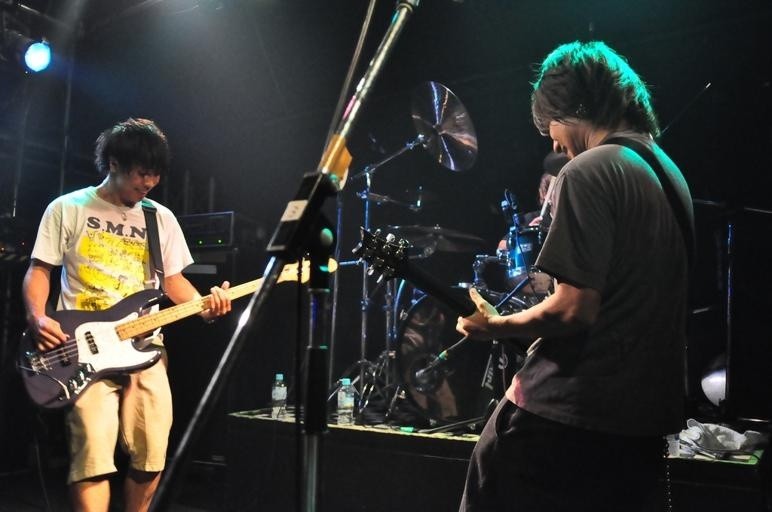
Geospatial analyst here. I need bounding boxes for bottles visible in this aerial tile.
[270,373,288,421]
[337,378,355,427]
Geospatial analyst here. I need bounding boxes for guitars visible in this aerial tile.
[351,226,536,349]
[14,257,337,409]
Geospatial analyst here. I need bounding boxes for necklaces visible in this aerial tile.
[104,181,132,221]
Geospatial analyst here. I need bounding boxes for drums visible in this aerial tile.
[506,225,554,296]
[473,254,506,293]
[397,286,536,429]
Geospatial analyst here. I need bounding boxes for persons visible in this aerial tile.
[455,41,693,512]
[21,118,231,512]
[497,152,570,253]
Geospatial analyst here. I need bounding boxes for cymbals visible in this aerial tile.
[409,79,479,170]
[357,190,410,211]
[396,223,492,242]
